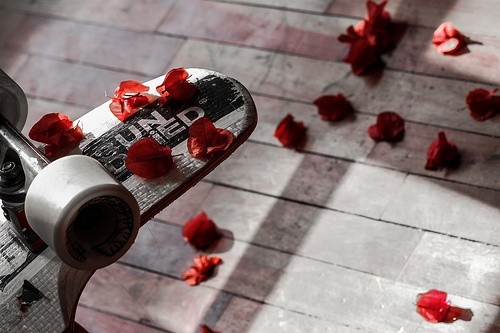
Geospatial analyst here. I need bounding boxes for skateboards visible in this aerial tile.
[0,67,258,333]
